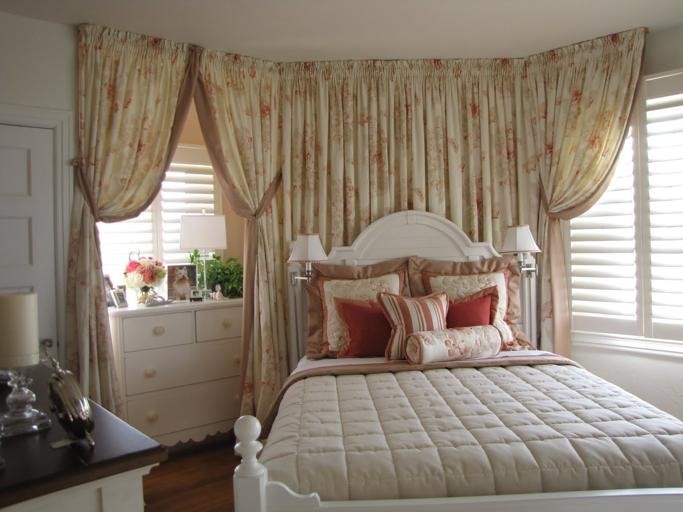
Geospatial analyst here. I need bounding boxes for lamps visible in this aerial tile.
[499,224,542,278]
[180,209,226,300]
[286,234,328,287]
[0,292,52,438]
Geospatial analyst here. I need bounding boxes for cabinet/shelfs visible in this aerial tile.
[107,297,244,460]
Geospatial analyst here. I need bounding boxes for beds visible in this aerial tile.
[233,210,683,512]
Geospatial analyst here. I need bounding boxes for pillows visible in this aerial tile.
[446,285,499,329]
[407,256,535,350]
[307,257,409,360]
[404,324,504,363]
[376,289,449,362]
[334,297,392,360]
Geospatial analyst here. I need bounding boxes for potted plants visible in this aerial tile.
[189,249,243,298]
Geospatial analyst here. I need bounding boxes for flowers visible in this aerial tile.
[124,257,165,293]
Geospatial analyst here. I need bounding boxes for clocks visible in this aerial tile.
[37,345,96,452]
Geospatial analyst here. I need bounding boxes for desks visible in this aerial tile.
[0,362,167,512]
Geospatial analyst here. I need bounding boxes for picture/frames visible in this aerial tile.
[167,264,198,302]
[104,276,128,308]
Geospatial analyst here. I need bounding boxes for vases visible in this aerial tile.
[136,289,152,303]
